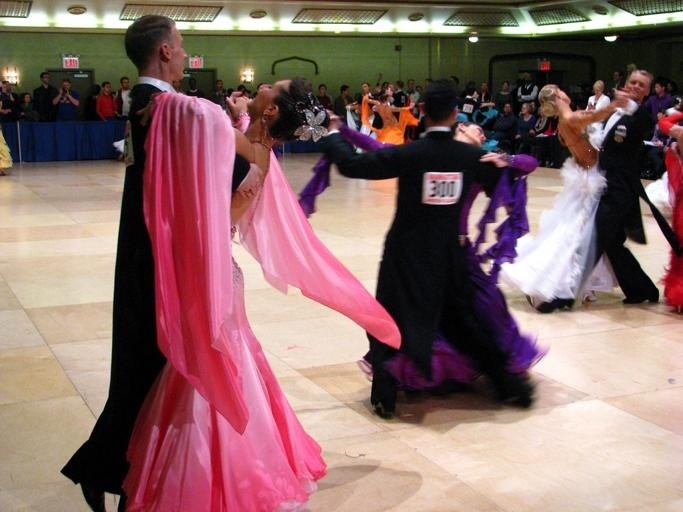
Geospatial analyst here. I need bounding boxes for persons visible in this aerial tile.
[1,65,683,174]
[61,15,250,512]
[123,78,400,512]
[298,81,550,420]
[504,72,683,314]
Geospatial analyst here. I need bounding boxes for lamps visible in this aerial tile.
[604,34,619,42]
[468,32,478,42]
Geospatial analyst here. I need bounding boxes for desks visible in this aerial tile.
[2,120,127,162]
[276,135,339,153]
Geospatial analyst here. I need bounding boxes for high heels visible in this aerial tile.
[582,292,598,304]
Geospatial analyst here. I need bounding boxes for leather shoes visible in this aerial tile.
[61,451,107,512]
[494,389,535,411]
[621,289,659,306]
[373,399,395,421]
[535,298,575,314]
